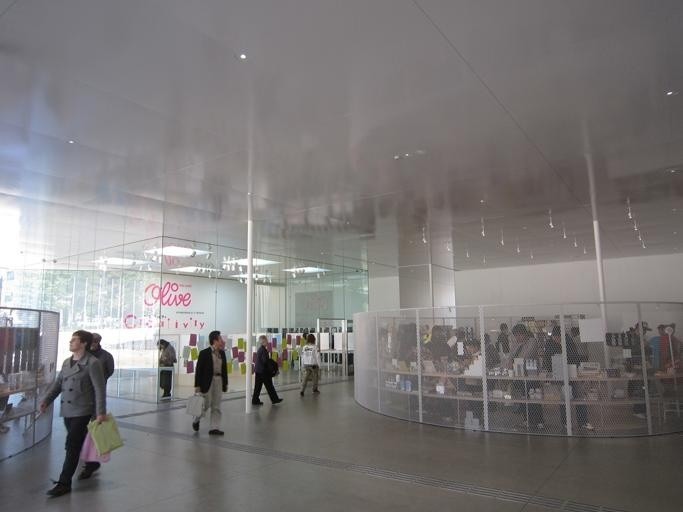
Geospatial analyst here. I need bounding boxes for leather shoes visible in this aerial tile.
[209,430,223,435]
[192,421,198,431]
[272,399,282,404]
[252,401,262,404]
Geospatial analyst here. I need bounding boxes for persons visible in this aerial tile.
[157,339,177,400]
[38,330,108,497]
[88,333,114,422]
[192,331,228,436]
[377,317,593,430]
[252,335,284,406]
[299,334,321,395]
[622,323,683,419]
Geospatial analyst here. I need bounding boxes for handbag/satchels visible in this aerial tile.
[86,413,124,456]
[186,392,205,418]
[80,432,110,463]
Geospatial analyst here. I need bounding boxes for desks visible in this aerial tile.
[114,365,175,404]
[286,349,353,372]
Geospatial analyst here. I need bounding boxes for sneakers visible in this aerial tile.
[632,412,646,419]
[311,390,319,394]
[514,419,593,430]
[160,393,171,400]
[301,391,304,397]
[78,463,99,480]
[45,482,71,495]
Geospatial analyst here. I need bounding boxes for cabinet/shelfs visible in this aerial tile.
[0,376,53,437]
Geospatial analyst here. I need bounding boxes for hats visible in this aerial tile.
[636,322,650,331]
